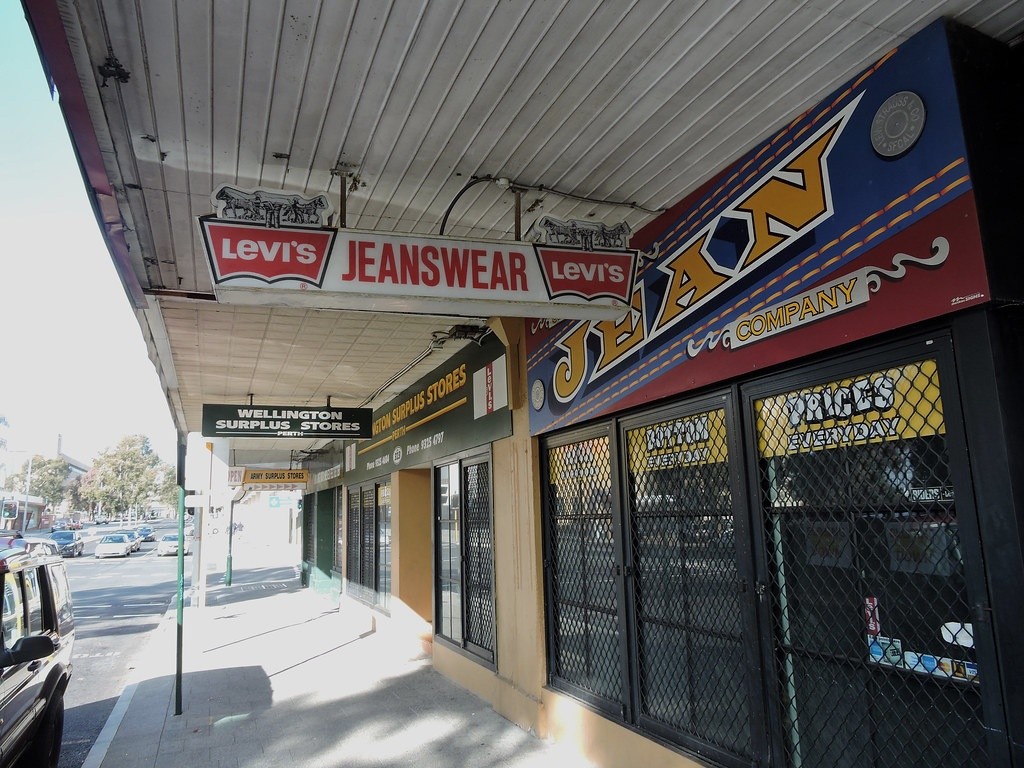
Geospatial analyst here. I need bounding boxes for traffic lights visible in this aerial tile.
[2,501,18,519]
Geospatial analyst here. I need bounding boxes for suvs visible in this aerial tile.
[0,547,76,768]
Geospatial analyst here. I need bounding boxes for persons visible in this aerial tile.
[16,530,22,538]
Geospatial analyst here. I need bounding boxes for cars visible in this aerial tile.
[50,518,84,533]
[148,513,156,519]
[49,531,84,558]
[133,524,156,542]
[664,530,738,589]
[183,526,194,536]
[156,534,191,557]
[124,516,135,521]
[95,534,131,559]
[111,530,142,552]
[113,516,120,522]
[95,516,109,525]
